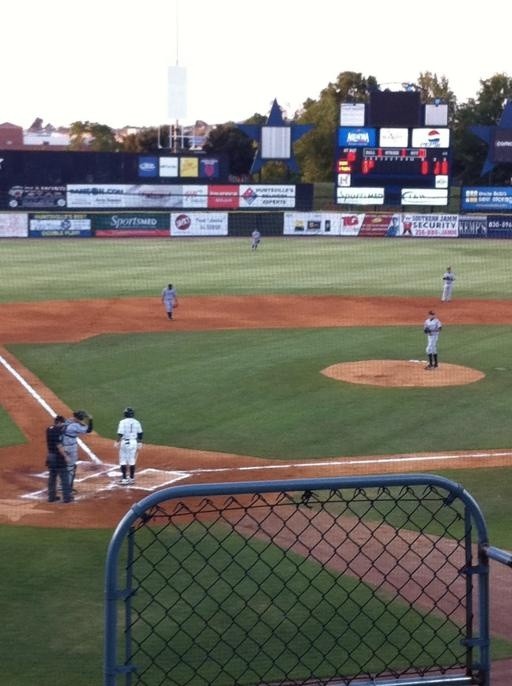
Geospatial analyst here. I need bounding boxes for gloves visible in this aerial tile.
[113,442,119,449]
[137,443,143,450]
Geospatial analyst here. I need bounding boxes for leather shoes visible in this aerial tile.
[48,497,60,502]
[64,496,74,503]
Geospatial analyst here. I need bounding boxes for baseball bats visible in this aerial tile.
[135,449,139,464]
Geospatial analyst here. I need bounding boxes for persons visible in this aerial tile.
[161,284,180,320]
[249,226,261,252]
[401,213,412,236]
[58,410,95,496]
[386,216,400,238]
[45,414,75,504]
[111,406,144,485]
[440,265,456,303]
[423,309,442,370]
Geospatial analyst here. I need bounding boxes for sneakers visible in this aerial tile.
[434,364,439,369]
[128,479,135,484]
[424,364,433,370]
[117,479,128,486]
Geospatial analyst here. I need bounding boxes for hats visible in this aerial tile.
[55,415,69,426]
[429,310,435,315]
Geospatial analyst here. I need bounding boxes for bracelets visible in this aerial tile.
[62,454,68,459]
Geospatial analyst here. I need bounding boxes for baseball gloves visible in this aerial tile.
[172,302,179,309]
[425,327,430,331]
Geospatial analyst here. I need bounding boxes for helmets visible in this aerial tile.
[123,407,135,418]
[73,409,89,420]
